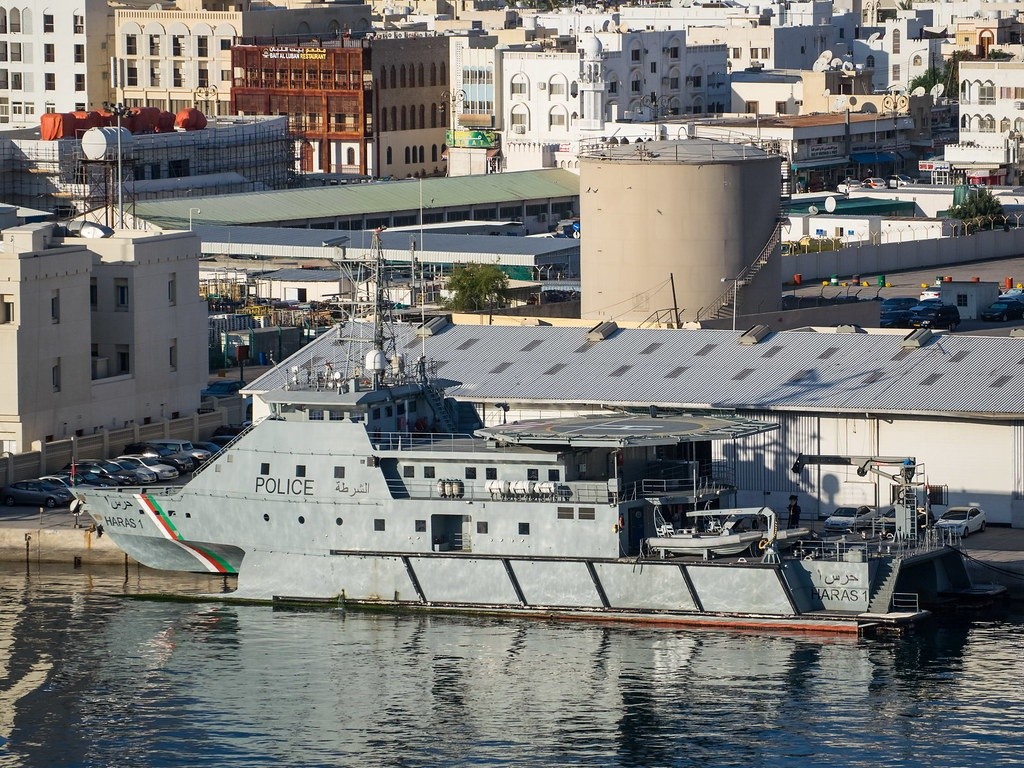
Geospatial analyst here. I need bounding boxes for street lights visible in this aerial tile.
[437,88,468,146]
[639,94,673,141]
[194,85,221,117]
[879,90,909,189]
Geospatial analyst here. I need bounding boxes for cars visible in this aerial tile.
[934,507,986,539]
[824,505,877,533]
[836,174,917,196]
[981,288,1024,322]
[0,421,252,509]
[874,507,935,539]
[200,380,247,403]
[880,286,962,329]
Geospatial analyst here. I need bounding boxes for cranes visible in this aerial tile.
[790,452,925,541]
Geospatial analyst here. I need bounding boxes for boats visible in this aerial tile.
[67,224,976,643]
[645,531,763,555]
